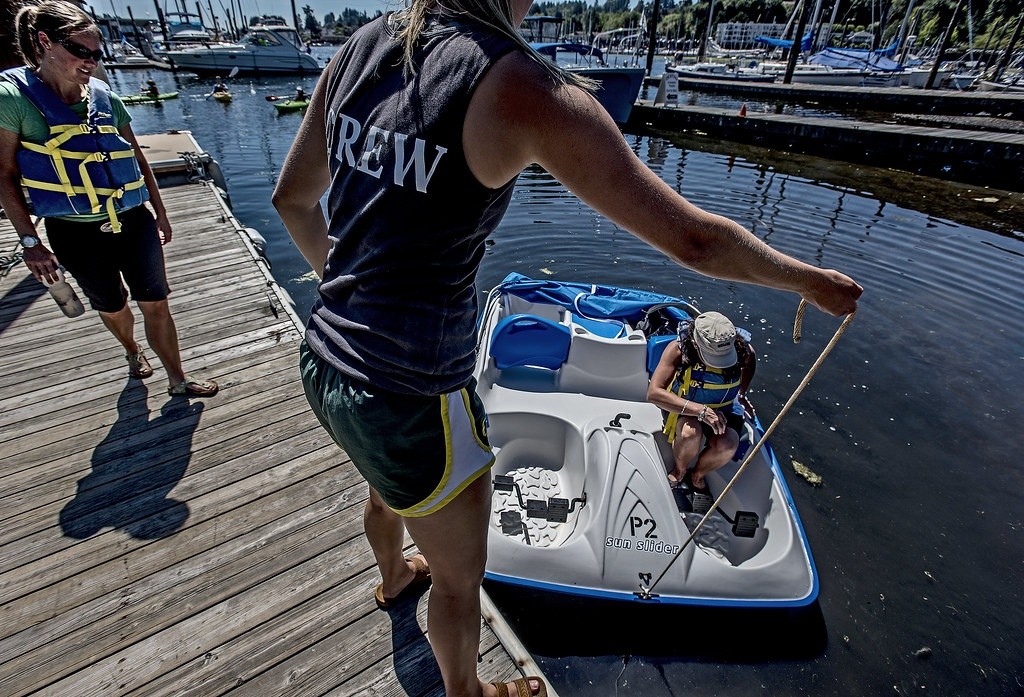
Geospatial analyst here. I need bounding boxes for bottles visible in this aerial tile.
[38,261,87,320]
[740,104,746,116]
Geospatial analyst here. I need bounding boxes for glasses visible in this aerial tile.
[61,40,104,60]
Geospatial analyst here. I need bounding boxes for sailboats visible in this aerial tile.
[664,0,1024,91]
[118,0,312,62]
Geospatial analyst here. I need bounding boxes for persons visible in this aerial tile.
[0,0,221,398]
[212,76,229,93]
[253,34,269,46]
[293,86,309,102]
[270,0,864,697]
[646,312,756,490]
[141,79,159,97]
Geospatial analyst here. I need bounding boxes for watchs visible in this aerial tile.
[20,234,40,247]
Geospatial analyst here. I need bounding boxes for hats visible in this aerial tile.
[693,311,737,368]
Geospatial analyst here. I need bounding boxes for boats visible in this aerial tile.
[211,91,234,103]
[163,27,327,76]
[273,97,310,112]
[120,91,179,103]
[516,16,646,125]
[472,271,821,609]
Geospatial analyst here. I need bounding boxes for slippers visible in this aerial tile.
[689,471,710,500]
[491,676,547,697]
[375,550,431,606]
[125,343,152,379]
[167,375,219,396]
[667,476,685,488]
